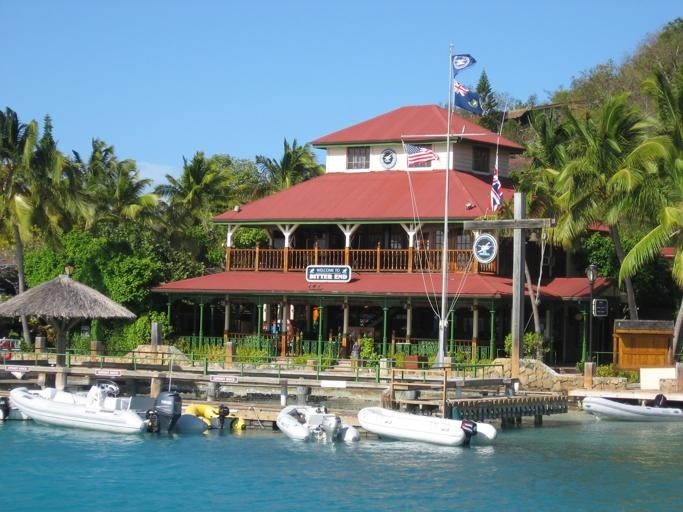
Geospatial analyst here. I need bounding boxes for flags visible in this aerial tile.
[490,135,505,213]
[453,79,485,114]
[405,143,440,167]
[452,54,477,79]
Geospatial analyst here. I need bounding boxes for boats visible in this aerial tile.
[275,405,361,447]
[358,407,498,445]
[358,436,496,460]
[0,397,29,422]
[8,381,208,436]
[186,403,245,434]
[582,393,682,425]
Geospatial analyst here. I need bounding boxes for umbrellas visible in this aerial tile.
[0,268,137,390]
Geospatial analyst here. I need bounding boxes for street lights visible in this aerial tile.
[584,263,597,379]
[64,263,74,352]
